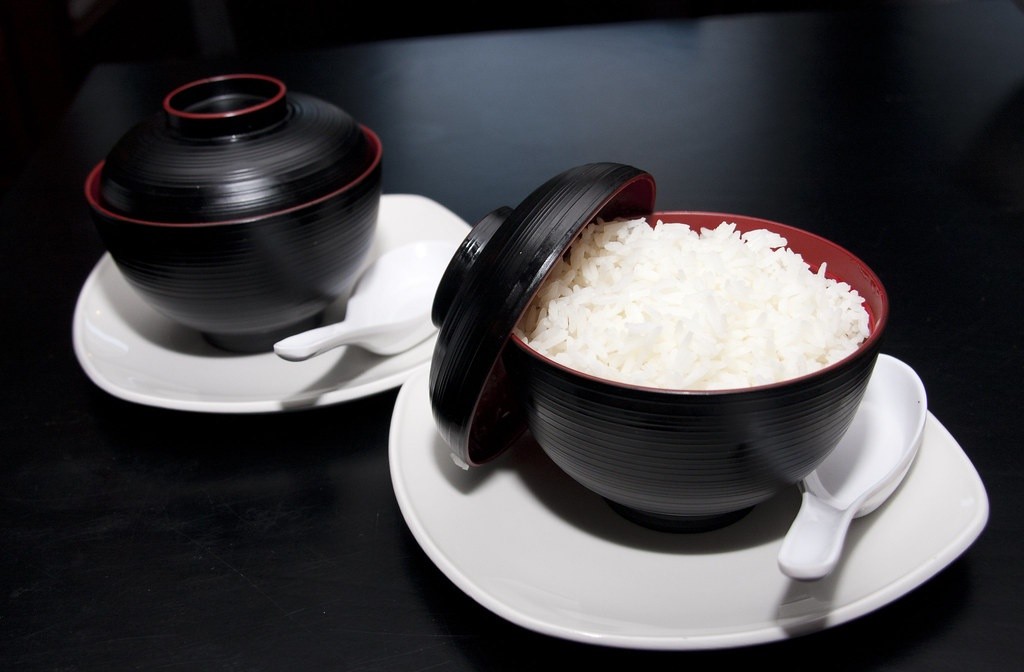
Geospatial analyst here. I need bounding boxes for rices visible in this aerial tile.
[511,211,871,392]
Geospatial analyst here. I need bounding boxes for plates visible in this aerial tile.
[75,194,478,410]
[388,359,992,650]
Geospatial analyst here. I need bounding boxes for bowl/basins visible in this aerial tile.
[85,71,381,343]
[507,210,887,518]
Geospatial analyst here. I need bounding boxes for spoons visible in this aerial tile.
[272,242,454,360]
[777,352,929,579]
